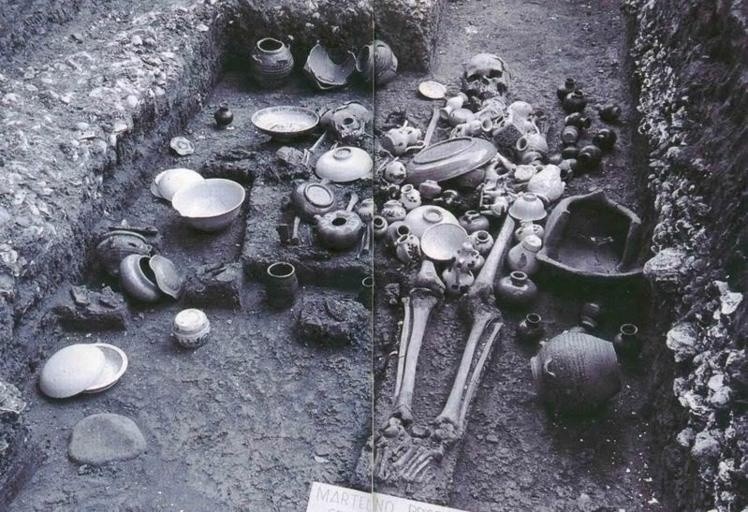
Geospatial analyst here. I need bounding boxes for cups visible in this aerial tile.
[267,262,299,308]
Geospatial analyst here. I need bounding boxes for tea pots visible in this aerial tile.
[382,120,427,155]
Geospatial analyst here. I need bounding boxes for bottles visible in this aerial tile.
[247,37,296,91]
[360,162,443,310]
[354,38,400,86]
[213,101,233,131]
[443,77,641,409]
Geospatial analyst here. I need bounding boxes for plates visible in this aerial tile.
[407,137,501,185]
[418,80,445,99]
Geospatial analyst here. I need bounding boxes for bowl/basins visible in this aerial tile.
[151,167,203,201]
[82,342,129,393]
[94,230,150,277]
[170,179,246,234]
[41,343,104,398]
[314,146,372,183]
[120,254,162,303]
[295,182,338,222]
[423,220,471,262]
[403,203,458,241]
[251,105,320,141]
